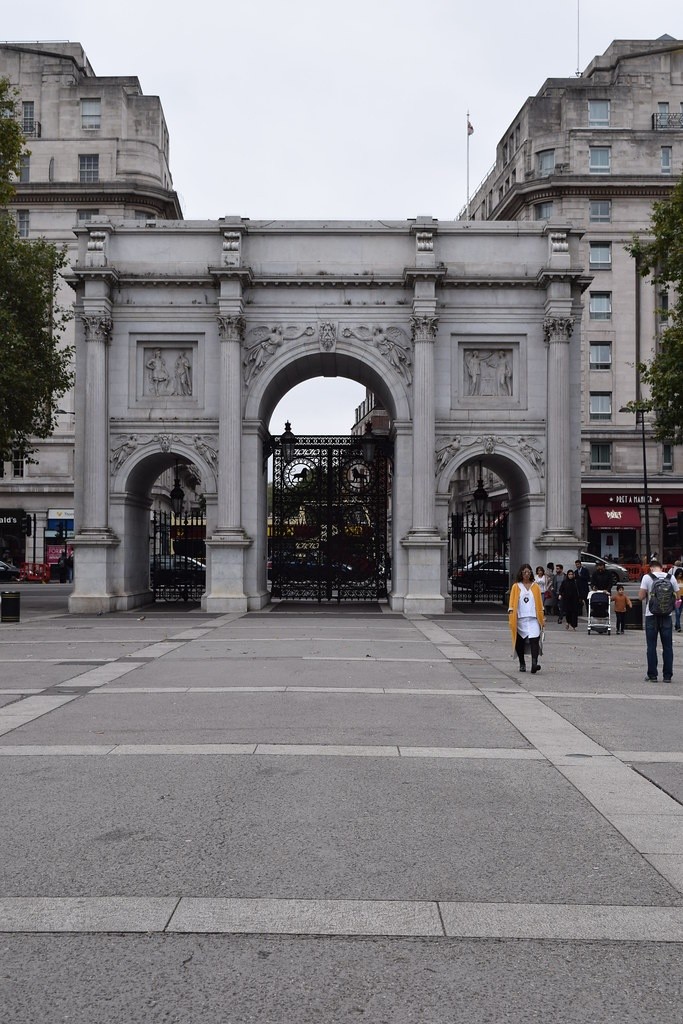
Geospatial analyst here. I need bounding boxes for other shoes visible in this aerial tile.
[663,677,671,683]
[531,665,540,673]
[676,628,681,632]
[566,622,568,629]
[616,628,621,635]
[557,618,562,624]
[572,627,575,632]
[621,627,624,634]
[644,674,657,682]
[519,664,526,671]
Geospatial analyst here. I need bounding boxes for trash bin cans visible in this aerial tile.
[624,599,644,628]
[1,591,21,622]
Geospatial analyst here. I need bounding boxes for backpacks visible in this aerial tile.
[648,572,675,616]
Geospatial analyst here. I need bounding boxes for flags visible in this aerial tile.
[468,121,475,137]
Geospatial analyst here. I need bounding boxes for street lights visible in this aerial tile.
[618,406,654,565]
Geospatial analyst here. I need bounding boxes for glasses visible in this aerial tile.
[521,570,531,574]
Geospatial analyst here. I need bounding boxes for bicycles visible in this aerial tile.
[159,563,205,605]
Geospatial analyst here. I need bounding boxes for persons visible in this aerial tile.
[590,562,613,635]
[482,435,497,454]
[502,436,543,475]
[349,325,411,386]
[469,350,483,395]
[535,560,591,632]
[435,434,461,476]
[508,563,547,673]
[489,349,511,396]
[319,321,336,351]
[159,434,170,451]
[604,552,683,683]
[611,586,632,634]
[110,434,152,475]
[174,351,191,396]
[244,324,308,386]
[477,551,501,560]
[193,434,219,477]
[146,349,167,396]
[58,549,74,583]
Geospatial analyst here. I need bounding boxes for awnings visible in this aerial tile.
[588,507,683,530]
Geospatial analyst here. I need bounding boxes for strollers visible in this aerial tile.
[586,589,612,636]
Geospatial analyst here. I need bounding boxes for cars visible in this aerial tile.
[0,559,20,582]
[449,558,509,593]
[581,551,630,587]
[150,554,207,587]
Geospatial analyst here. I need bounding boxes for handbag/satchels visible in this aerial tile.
[675,599,682,608]
[545,591,551,599]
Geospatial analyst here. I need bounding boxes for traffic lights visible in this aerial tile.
[22,514,32,537]
[54,521,64,541]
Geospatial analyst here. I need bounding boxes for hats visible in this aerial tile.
[595,560,607,565]
